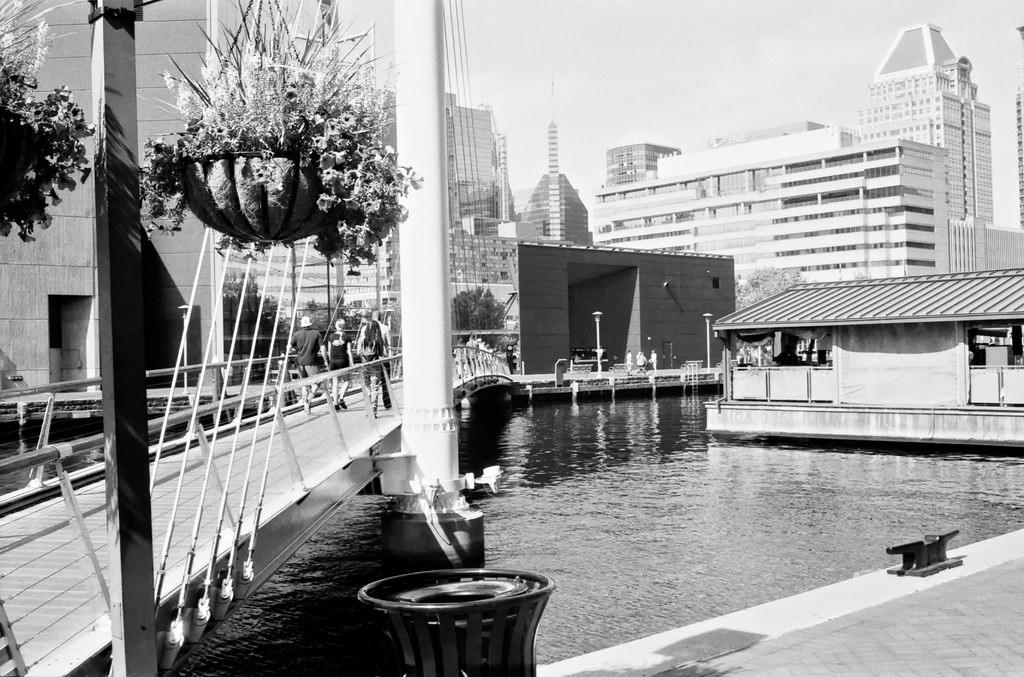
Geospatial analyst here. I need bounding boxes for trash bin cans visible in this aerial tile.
[357,568,557,677]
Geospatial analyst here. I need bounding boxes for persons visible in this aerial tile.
[465,336,479,376]
[626,351,633,376]
[290,316,328,415]
[327,318,353,410]
[772,345,798,367]
[358,320,383,418]
[454,338,465,377]
[636,352,647,372]
[356,311,392,410]
[476,338,493,373]
[651,350,657,373]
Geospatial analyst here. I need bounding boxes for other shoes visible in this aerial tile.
[365,414,378,419]
[304,399,312,416]
[338,402,348,409]
[335,404,341,411]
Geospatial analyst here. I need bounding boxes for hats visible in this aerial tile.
[300,316,314,329]
